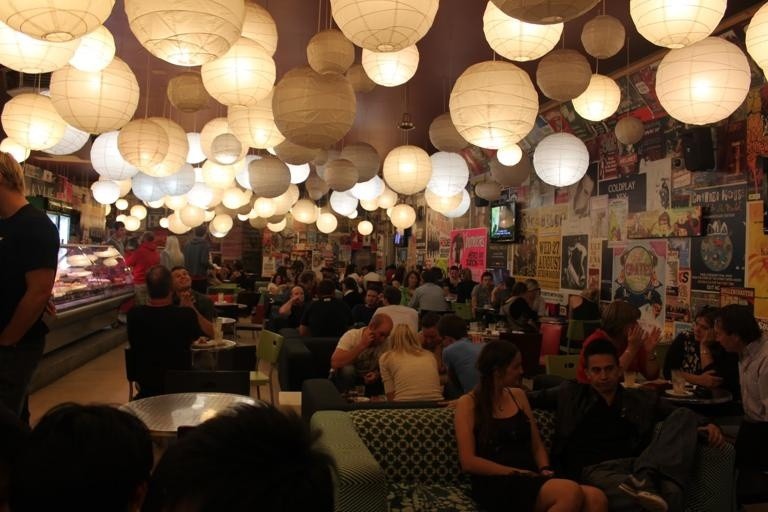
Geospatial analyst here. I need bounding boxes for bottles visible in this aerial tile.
[488,308,495,331]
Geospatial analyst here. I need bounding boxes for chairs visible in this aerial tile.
[124,345,136,402]
[250,330,284,407]
[452,302,473,321]
[560,319,603,355]
[544,354,580,383]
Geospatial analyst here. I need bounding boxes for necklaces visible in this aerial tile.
[492,394,508,416]
[699,348,711,355]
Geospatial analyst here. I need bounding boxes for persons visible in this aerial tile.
[384,264,395,287]
[29,404,151,512]
[578,300,663,391]
[342,278,364,305]
[443,264,460,290]
[525,343,725,512]
[140,401,341,512]
[521,277,548,315]
[351,287,384,324]
[329,311,393,397]
[379,323,446,400]
[109,221,128,248]
[668,304,728,393]
[276,256,303,278]
[321,255,336,282]
[421,319,451,371]
[493,276,515,305]
[413,264,424,285]
[117,229,162,303]
[186,225,211,281]
[449,342,607,512]
[162,237,187,276]
[431,266,448,288]
[438,314,492,399]
[363,264,383,290]
[568,285,604,346]
[264,270,290,296]
[471,270,496,317]
[0,147,61,512]
[167,266,218,343]
[502,285,527,326]
[457,267,480,303]
[715,301,768,510]
[371,285,418,338]
[399,271,418,306]
[271,286,306,327]
[391,265,405,285]
[307,280,351,337]
[232,263,246,286]
[409,270,446,313]
[128,265,206,396]
[298,267,318,296]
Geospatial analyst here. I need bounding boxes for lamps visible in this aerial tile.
[475,181,501,201]
[286,162,310,184]
[324,159,359,192]
[202,159,236,189]
[254,197,277,218]
[235,155,262,190]
[745,2,768,83]
[39,91,90,156]
[205,192,222,209]
[497,144,523,166]
[358,221,373,236]
[630,0,728,49]
[339,142,381,184]
[360,199,378,211]
[449,60,539,150]
[249,157,291,198]
[330,190,359,216]
[425,187,464,211]
[244,190,253,205]
[615,117,644,145]
[345,62,376,94]
[383,145,432,196]
[227,85,276,148]
[386,207,394,217]
[213,214,234,232]
[348,209,358,219]
[168,214,188,235]
[581,14,625,59]
[655,36,752,126]
[305,173,330,201]
[185,132,206,164]
[267,214,285,224]
[429,112,470,153]
[249,216,267,229]
[125,216,141,232]
[306,28,355,74]
[155,164,196,197]
[316,213,338,234]
[164,195,187,211]
[193,168,203,183]
[536,48,593,101]
[187,183,215,208]
[265,126,286,156]
[0,0,116,43]
[268,217,287,233]
[90,130,139,181]
[390,204,417,230]
[49,56,140,135]
[116,214,127,225]
[1,92,66,151]
[271,65,357,149]
[200,117,249,165]
[139,117,190,178]
[220,203,236,219]
[287,184,300,206]
[130,204,147,220]
[273,139,322,165]
[490,151,530,187]
[249,209,257,219]
[362,43,420,87]
[237,213,249,221]
[209,220,229,238]
[483,1,564,62]
[305,205,319,224]
[572,74,621,122]
[115,199,128,210]
[292,199,314,222]
[238,203,251,215]
[311,149,328,165]
[93,180,120,204]
[330,0,440,53]
[0,137,31,163]
[148,200,164,209]
[201,36,277,110]
[198,208,216,222]
[221,187,244,209]
[490,0,601,25]
[124,0,246,67]
[316,150,342,182]
[159,217,168,228]
[132,172,164,202]
[533,132,590,187]
[350,176,385,200]
[101,204,111,216]
[242,2,279,57]
[378,184,397,209]
[0,20,81,74]
[439,188,471,218]
[69,25,116,72]
[428,150,470,198]
[117,118,169,169]
[167,72,209,114]
[179,204,205,228]
[211,133,242,165]
[99,175,132,197]
[274,190,292,215]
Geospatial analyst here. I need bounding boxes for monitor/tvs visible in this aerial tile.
[490,202,517,243]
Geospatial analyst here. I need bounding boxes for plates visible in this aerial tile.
[665,389,693,396]
[620,383,641,388]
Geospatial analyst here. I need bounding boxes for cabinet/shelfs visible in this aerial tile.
[29,245,134,393]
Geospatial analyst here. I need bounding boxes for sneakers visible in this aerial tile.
[619,473,668,511]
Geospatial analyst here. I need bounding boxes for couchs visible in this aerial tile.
[301,378,448,426]
[311,407,556,512]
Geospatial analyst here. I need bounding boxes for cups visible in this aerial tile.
[212,317,223,334]
[214,332,224,348]
[624,371,636,387]
[470,322,477,332]
[489,324,495,334]
[672,371,686,394]
[218,293,224,304]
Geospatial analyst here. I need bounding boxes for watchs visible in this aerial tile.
[539,465,552,471]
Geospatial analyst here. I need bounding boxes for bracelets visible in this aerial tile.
[625,349,634,360]
